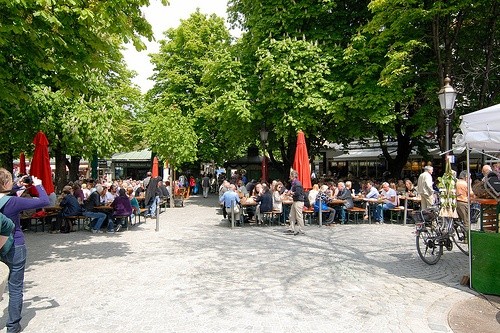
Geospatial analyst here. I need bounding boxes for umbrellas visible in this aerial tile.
[293,130,313,191]
[19,152,27,175]
[29,129,55,195]
[151,157,158,178]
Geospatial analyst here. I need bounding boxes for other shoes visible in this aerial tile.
[294,231,304,236]
[116,225,122,232]
[48,229,61,234]
[105,229,115,233]
[84,225,92,231]
[91,229,104,234]
[283,229,294,233]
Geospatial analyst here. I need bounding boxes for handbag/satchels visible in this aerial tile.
[410,207,436,222]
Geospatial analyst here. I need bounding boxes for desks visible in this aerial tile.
[397,195,421,201]
[357,197,380,222]
[44,205,64,232]
[240,197,259,225]
[94,204,114,227]
[332,198,347,222]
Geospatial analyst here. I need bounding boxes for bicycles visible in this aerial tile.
[414,207,469,265]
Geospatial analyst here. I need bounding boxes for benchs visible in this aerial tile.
[20,209,149,232]
[263,206,416,226]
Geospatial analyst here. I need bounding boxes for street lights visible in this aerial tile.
[258,120,269,183]
[436,73,459,174]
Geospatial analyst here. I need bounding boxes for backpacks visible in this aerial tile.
[0,195,16,258]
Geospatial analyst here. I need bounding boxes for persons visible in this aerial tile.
[440,163,500,244]
[418,166,435,211]
[218,168,418,225]
[286,169,306,235]
[10,168,188,234]
[0,167,51,333]
[190,171,227,198]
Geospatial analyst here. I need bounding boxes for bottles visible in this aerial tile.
[49,192,56,206]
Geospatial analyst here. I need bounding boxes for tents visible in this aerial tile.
[453,104,500,289]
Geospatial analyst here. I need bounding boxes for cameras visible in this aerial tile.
[23,176,33,183]
